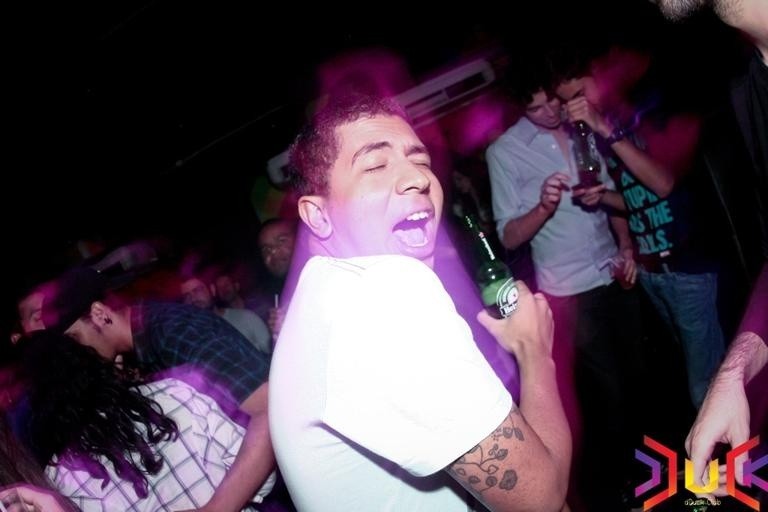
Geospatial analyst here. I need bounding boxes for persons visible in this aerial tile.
[485,77,638,452]
[545,42,725,411]
[269,90,575,512]
[655,0,768,507]
[275,70,486,328]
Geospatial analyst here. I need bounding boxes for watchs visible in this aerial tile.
[604,128,623,149]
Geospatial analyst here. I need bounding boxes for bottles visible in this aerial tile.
[569,121,600,192]
[462,215,519,319]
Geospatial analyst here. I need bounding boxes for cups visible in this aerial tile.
[0,487,27,511]
